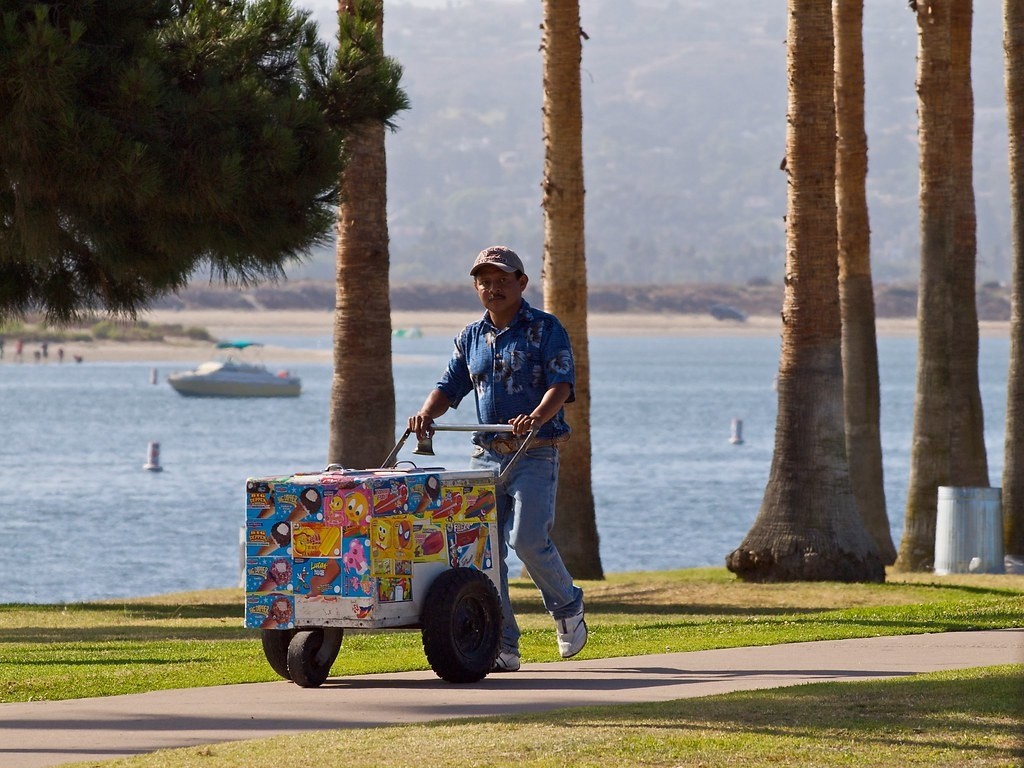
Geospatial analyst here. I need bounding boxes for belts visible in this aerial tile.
[492,434,570,454]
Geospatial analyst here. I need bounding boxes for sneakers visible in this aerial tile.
[555,599,588,657]
[490,652,520,672]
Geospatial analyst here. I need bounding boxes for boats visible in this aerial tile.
[165,341,305,400]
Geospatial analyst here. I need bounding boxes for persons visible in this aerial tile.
[0,338,83,363]
[408,247,588,671]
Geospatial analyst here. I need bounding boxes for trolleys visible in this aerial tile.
[241,421,539,689]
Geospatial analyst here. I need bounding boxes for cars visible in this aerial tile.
[709,304,745,324]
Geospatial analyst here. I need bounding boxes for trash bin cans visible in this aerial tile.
[933,487,1006,574]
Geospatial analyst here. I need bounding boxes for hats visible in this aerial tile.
[469,246,524,275]
